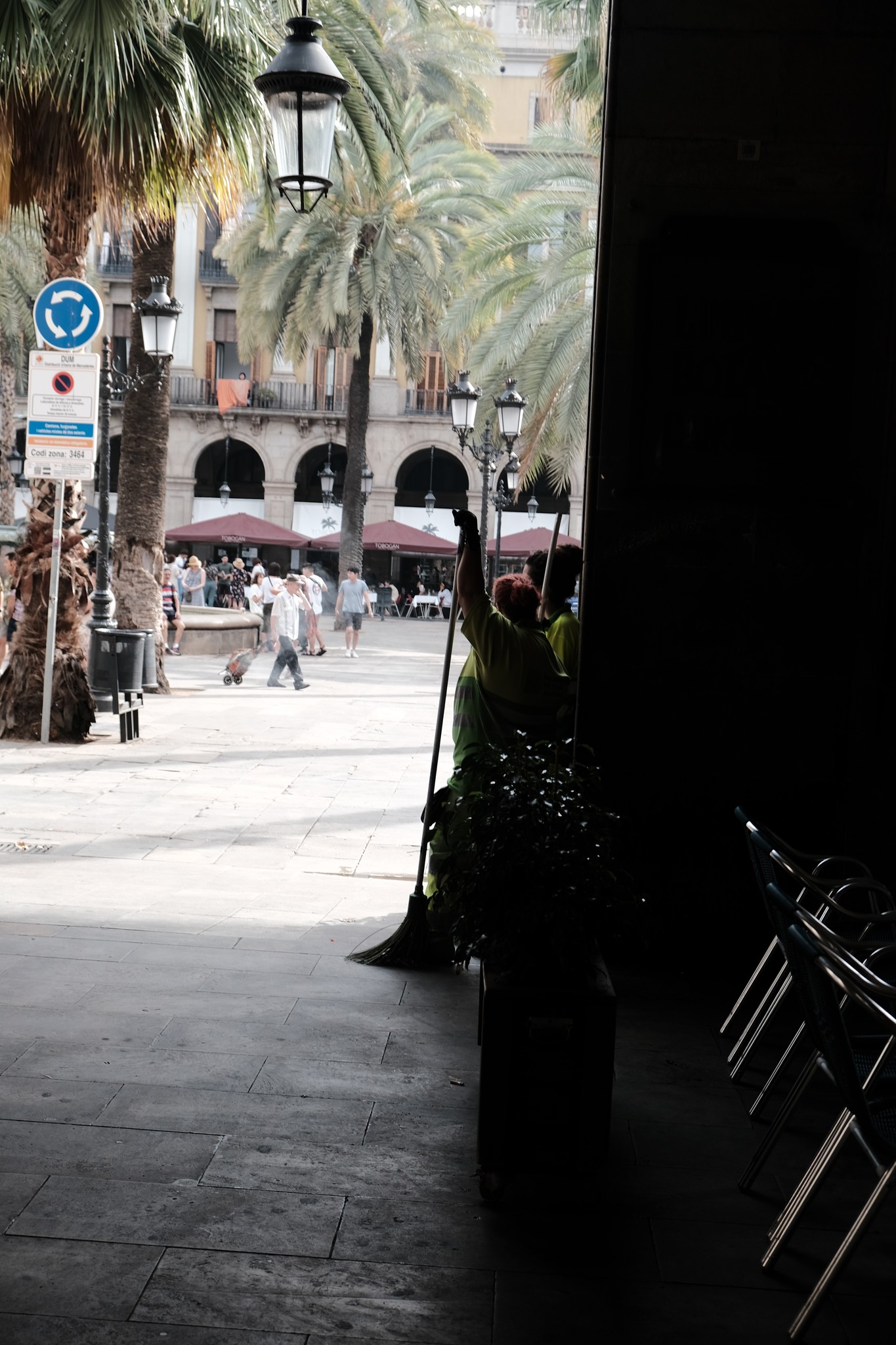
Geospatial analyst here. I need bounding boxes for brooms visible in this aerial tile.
[344,504,472,976]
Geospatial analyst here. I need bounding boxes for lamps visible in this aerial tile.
[527,483,539,523]
[253,0,352,214]
[219,434,231,507]
[317,432,337,512]
[424,444,436,517]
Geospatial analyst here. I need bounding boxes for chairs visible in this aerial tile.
[400,594,417,617]
[391,594,401,617]
[720,805,896,1338]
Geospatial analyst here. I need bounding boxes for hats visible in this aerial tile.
[286,573,305,585]
[187,555,199,566]
[233,558,245,570]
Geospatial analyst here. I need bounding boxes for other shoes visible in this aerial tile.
[170,646,181,655]
[293,680,310,690]
[267,679,286,687]
[345,647,350,657]
[351,650,358,658]
[165,647,172,655]
[298,648,308,653]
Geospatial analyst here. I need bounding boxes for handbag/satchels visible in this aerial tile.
[184,592,192,603]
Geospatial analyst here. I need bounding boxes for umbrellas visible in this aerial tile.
[161,511,309,562]
[309,520,457,598]
[479,525,584,558]
[10,498,117,535]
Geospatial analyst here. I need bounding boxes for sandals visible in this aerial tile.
[316,645,327,656]
[302,649,315,656]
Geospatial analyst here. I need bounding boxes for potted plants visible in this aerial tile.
[256,387,274,408]
[421,728,627,1202]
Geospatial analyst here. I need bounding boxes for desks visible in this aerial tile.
[363,593,380,616]
[405,594,445,619]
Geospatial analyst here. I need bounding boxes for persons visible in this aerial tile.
[0,550,21,674]
[406,578,425,618]
[160,548,399,658]
[266,573,312,691]
[334,566,374,658]
[433,581,452,618]
[237,372,256,407]
[181,555,206,607]
[423,506,585,920]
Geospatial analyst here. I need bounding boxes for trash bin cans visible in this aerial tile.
[90,627,146,694]
[136,628,159,688]
